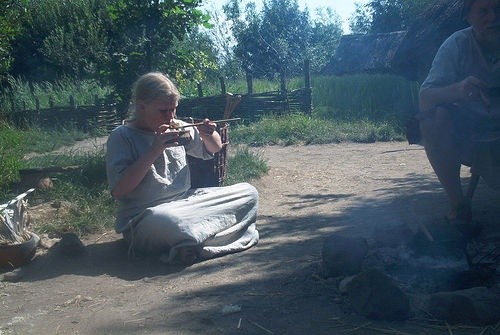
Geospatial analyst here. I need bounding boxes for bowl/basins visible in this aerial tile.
[480,87,500,104]
[162,130,192,145]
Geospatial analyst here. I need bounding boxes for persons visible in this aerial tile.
[105,72,259,272]
[416,1,500,230]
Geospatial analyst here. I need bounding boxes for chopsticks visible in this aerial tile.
[171,118,240,129]
